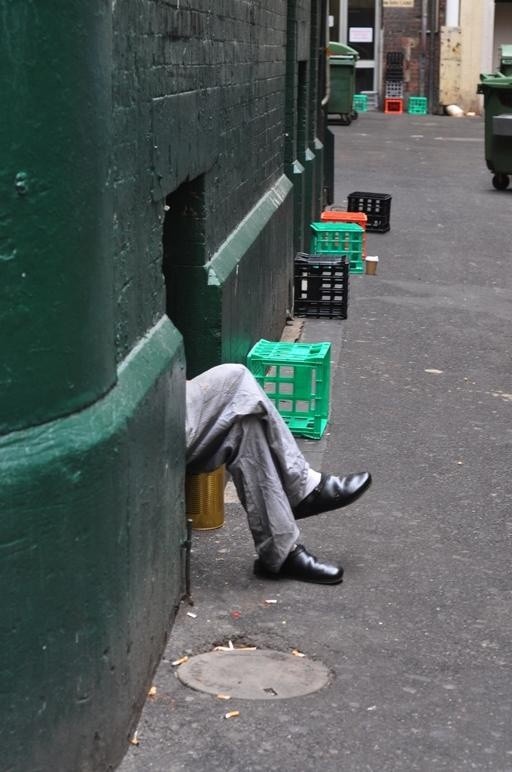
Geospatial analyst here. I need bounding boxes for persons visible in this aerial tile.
[185,362,371,584]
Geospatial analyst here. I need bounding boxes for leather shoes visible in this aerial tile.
[294,473,368,518]
[286,545,344,585]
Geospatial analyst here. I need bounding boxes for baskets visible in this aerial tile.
[354,94,368,112]
[293,250,348,321]
[348,192,392,233]
[383,53,404,114]
[312,210,368,275]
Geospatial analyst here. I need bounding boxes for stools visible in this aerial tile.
[384,51,404,115]
[292,191,392,319]
[353,91,379,112]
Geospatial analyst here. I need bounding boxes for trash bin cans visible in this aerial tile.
[497,43,512,76]
[477,72,512,189]
[327,41,360,125]
